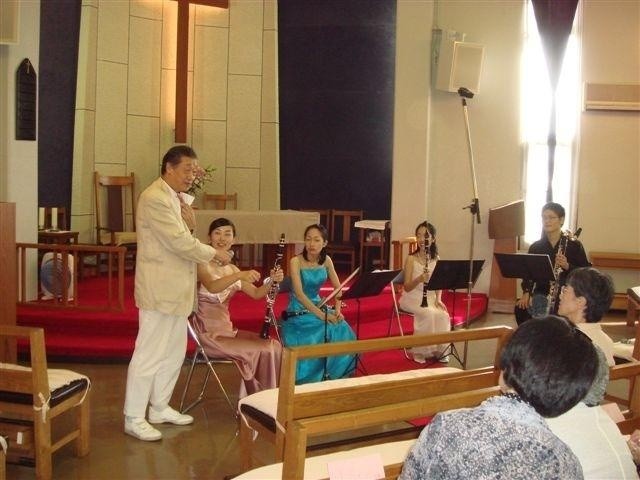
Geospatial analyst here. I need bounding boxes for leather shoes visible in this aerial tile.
[414,354,426,364]
[437,354,449,363]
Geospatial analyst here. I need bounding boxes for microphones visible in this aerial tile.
[458,87,474,99]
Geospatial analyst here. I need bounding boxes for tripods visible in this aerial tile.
[336,300,368,378]
[433,289,464,367]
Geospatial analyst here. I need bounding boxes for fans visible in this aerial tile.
[40,250,76,303]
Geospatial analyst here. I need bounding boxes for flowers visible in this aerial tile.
[186,164,217,196]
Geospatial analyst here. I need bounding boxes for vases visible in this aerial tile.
[180,192,195,205]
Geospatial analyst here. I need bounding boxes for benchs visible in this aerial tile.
[625,285,640,326]
[233,325,639,480]
[0,437,9,480]
[1,323,92,478]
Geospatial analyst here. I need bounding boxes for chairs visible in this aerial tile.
[320,209,364,275]
[177,309,241,419]
[92,170,137,279]
[385,270,437,362]
[202,192,237,211]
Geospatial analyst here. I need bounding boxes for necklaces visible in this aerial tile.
[417,251,432,265]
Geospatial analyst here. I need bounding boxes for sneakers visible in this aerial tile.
[148,406,194,426]
[124,416,163,442]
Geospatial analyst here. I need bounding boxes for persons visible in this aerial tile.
[398,223,451,364]
[192,218,284,399]
[397,315,599,480]
[515,203,589,326]
[558,267,616,367]
[279,224,358,384]
[123,146,234,441]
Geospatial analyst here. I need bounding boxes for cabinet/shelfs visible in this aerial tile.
[38,206,79,244]
[354,219,390,274]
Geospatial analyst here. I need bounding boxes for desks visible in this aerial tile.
[194,210,320,279]
[17,243,126,311]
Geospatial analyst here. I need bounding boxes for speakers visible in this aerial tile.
[436,40,486,95]
